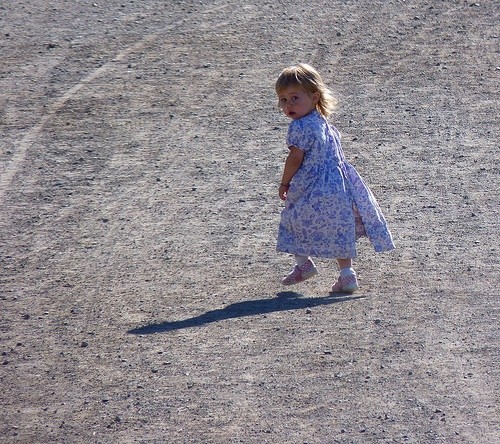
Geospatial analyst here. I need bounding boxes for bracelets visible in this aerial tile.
[281,183,289,187]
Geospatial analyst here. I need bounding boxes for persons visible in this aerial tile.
[275,63,396,293]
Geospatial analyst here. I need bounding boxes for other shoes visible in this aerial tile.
[332,274,359,293]
[281,259,319,285]
